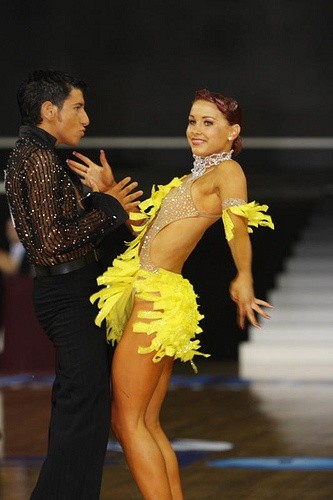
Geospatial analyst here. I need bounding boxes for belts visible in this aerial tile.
[35,249,99,278]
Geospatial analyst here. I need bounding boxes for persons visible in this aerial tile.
[67,87,274,500]
[5,79,143,500]
